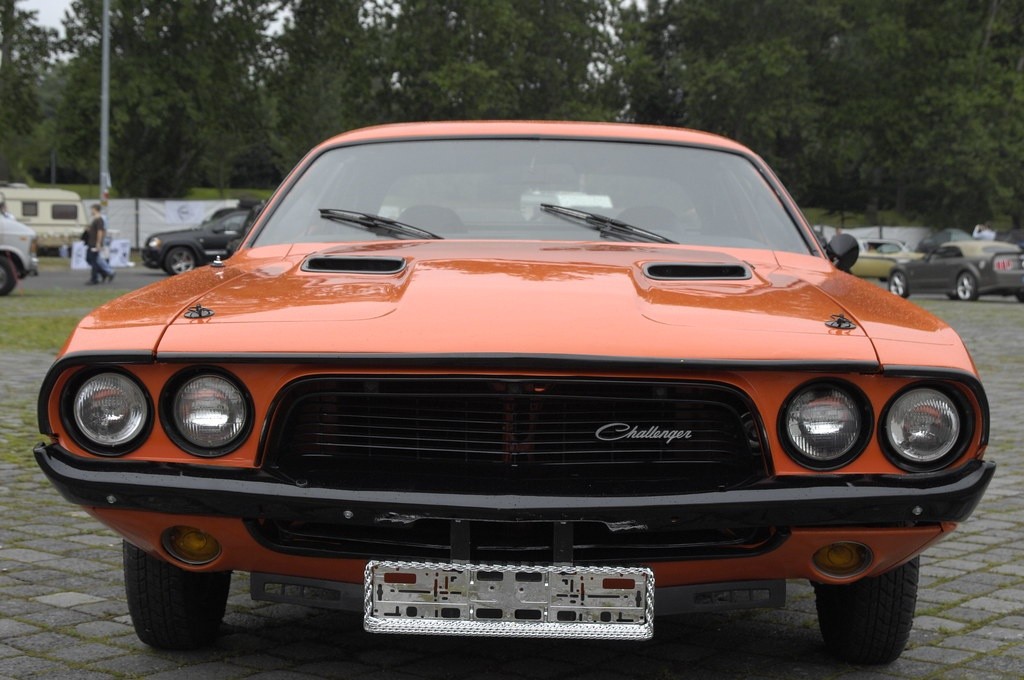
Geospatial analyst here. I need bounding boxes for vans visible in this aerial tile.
[0,181,90,258]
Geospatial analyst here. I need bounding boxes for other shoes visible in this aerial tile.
[85,281,99,285]
[110,272,116,282]
[102,272,108,283]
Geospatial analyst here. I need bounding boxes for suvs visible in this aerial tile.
[141,201,264,277]
[1,205,39,299]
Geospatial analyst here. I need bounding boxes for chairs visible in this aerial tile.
[614,204,686,242]
[393,204,468,236]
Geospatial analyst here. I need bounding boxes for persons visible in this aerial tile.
[972,222,996,241]
[85,204,115,285]
[831,227,842,238]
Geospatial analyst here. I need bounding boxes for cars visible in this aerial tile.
[849,236,936,282]
[887,237,1024,303]
[914,225,1024,257]
[31,118,999,666]
